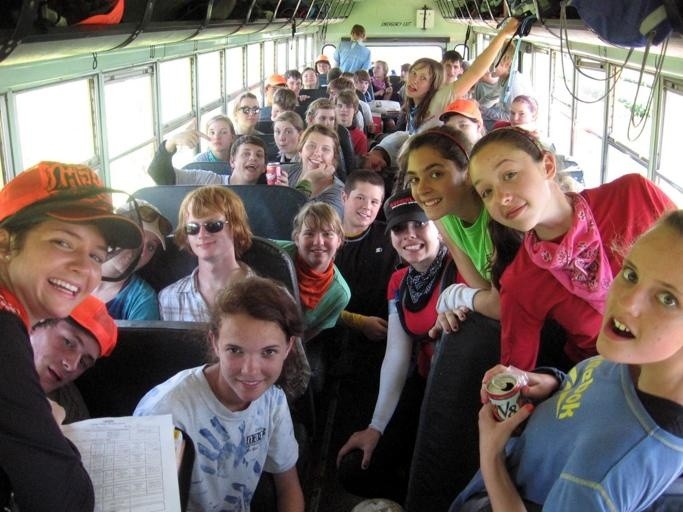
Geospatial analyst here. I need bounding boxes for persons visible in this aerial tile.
[0,17,683,512]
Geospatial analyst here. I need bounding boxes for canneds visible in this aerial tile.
[485,374,527,437]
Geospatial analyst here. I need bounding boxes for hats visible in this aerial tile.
[113,198,166,252]
[265,74,287,88]
[315,55,329,63]
[439,99,482,122]
[69,293,119,357]
[384,188,428,236]
[0,162,144,250]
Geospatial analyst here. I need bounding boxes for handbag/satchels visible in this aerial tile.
[573,0,672,46]
[480,0,570,20]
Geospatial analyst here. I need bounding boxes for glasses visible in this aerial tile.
[238,106,260,114]
[124,207,172,236]
[90,188,145,281]
[183,221,229,234]
